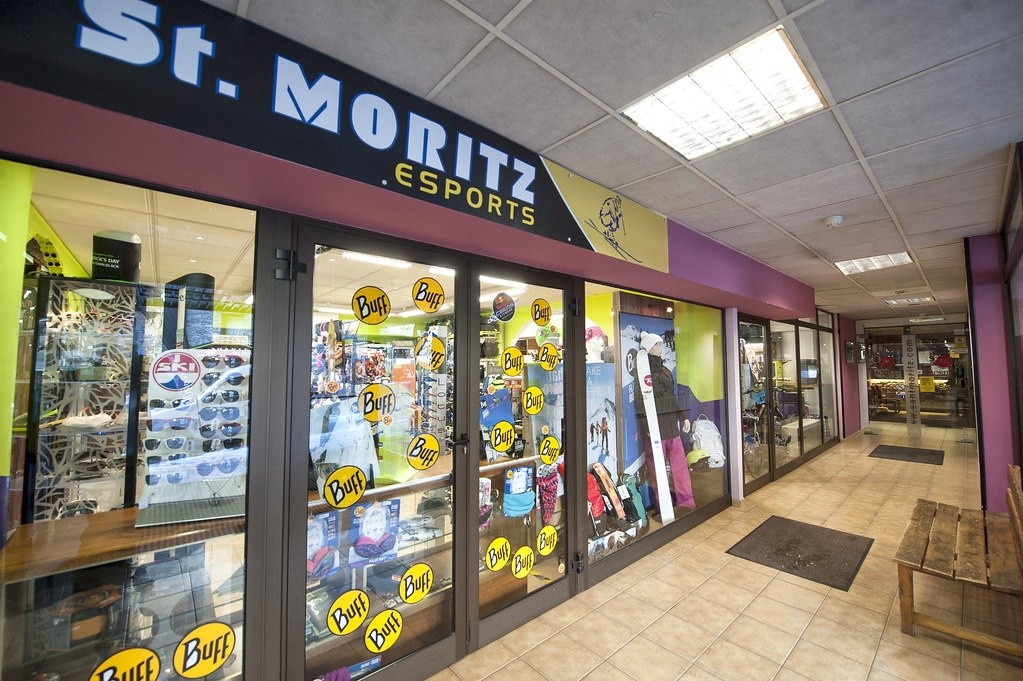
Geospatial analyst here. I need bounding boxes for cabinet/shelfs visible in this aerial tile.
[0,449,538,681]
[0,505,244,681]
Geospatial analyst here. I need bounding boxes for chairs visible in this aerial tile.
[870,385,901,416]
[890,462,1023,662]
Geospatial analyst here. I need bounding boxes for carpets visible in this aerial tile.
[867,444,945,467]
[725,515,875,592]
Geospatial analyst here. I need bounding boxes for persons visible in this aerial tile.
[634,332,695,508]
[590,423,596,442]
[600,417,611,454]
[596,421,601,446]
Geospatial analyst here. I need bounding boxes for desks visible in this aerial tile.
[783,383,818,391]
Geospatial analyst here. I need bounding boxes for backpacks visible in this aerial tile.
[585,474,608,537]
[690,413,726,468]
[618,473,650,539]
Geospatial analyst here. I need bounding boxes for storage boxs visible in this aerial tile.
[781,418,822,457]
[778,391,804,404]
[778,403,802,417]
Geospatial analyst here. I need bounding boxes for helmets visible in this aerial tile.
[686,448,710,468]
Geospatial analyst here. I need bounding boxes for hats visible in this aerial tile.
[639,330,662,352]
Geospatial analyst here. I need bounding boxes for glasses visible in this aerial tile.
[57,499,100,519]
[145,354,246,486]
